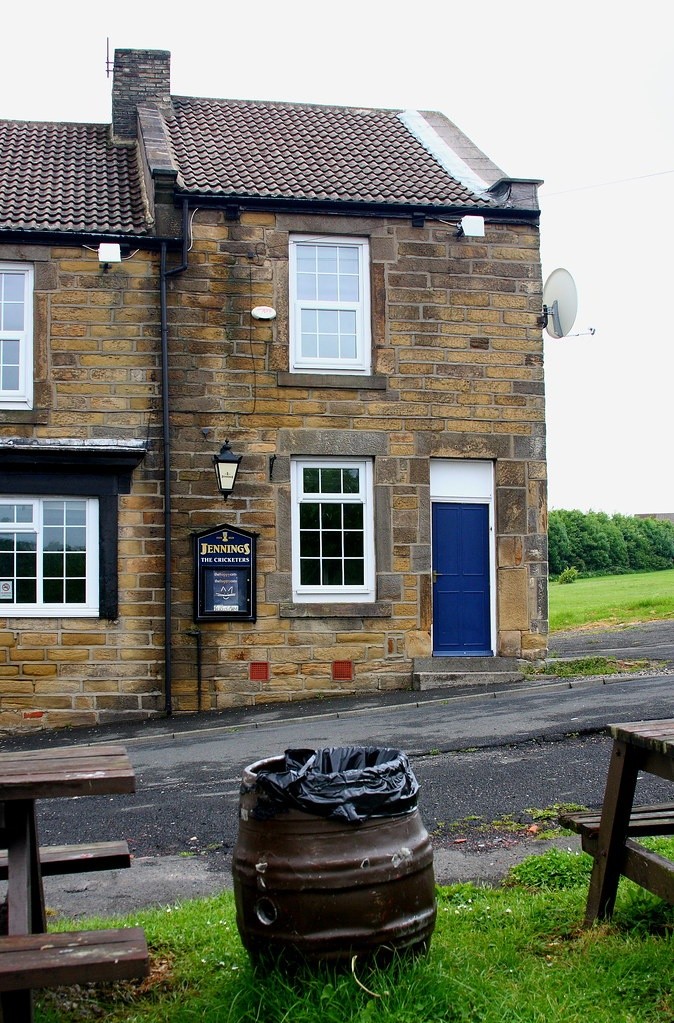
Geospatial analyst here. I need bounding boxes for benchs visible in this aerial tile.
[1,839,132,880]
[0,926,150,992]
[558,803,674,840]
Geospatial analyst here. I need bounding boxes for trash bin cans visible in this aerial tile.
[229,742,437,970]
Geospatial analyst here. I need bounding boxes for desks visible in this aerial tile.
[0,745,137,1023]
[582,719,674,932]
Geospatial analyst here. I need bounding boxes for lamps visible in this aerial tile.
[211,437,243,503]
[97,243,122,274]
[454,216,486,242]
